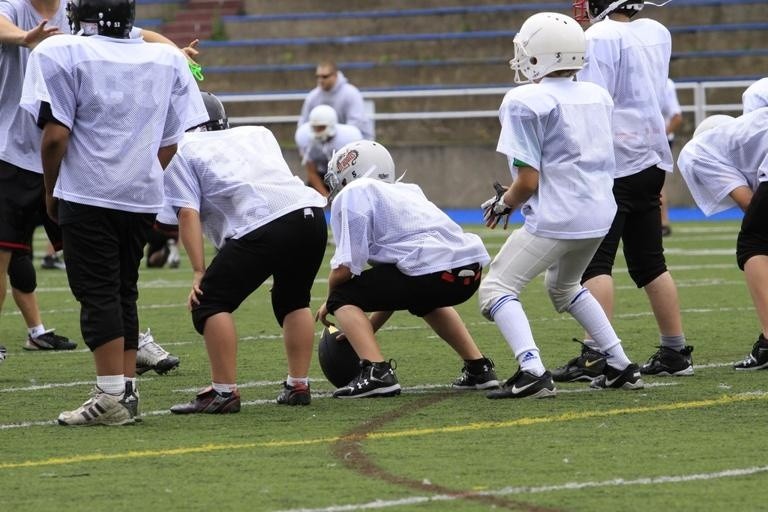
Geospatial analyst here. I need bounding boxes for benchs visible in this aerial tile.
[128,0,768,144]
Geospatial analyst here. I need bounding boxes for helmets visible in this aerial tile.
[66,0,136,39]
[200,90,228,130]
[509,12,586,84]
[324,141,394,202]
[572,0,644,22]
[307,105,338,141]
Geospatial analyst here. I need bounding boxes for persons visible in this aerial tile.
[657,76,683,236]
[313,138,500,401]
[475,10,648,401]
[17,0,212,429]
[674,106,767,374]
[544,0,693,384]
[163,86,328,416]
[294,61,374,244]
[40,199,180,270]
[0,0,200,377]
[741,74,768,116]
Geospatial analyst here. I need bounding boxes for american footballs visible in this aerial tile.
[318,325,362,388]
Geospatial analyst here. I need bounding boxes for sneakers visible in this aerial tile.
[58,389,141,428]
[487,365,556,398]
[171,386,242,414]
[25,328,78,350]
[136,327,179,377]
[275,381,312,404]
[552,345,608,382]
[451,358,500,390]
[639,345,695,377]
[589,362,644,390]
[41,254,66,269]
[333,358,402,399]
[732,333,767,371]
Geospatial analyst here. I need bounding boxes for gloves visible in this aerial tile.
[481,180,512,230]
